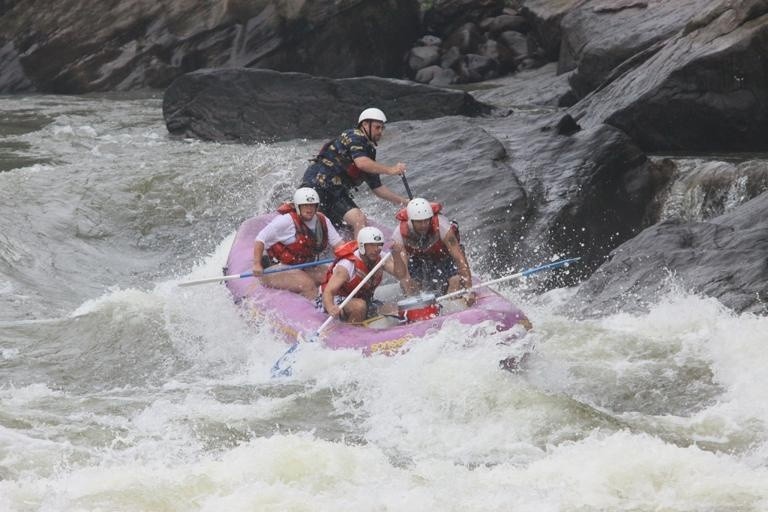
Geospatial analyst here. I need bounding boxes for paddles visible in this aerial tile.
[175,260,338,287]
[269,251,391,375]
[435,257,577,301]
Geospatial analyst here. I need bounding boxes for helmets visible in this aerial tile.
[406,197,434,233]
[357,226,385,255]
[358,107,387,123]
[293,187,321,216]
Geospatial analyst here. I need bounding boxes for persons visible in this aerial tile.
[322,226,407,323]
[391,197,476,307]
[252,187,345,301]
[300,107,410,240]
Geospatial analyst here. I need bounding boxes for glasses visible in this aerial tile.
[376,126,385,130]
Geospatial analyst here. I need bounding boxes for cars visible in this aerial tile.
[224,212,535,370]
